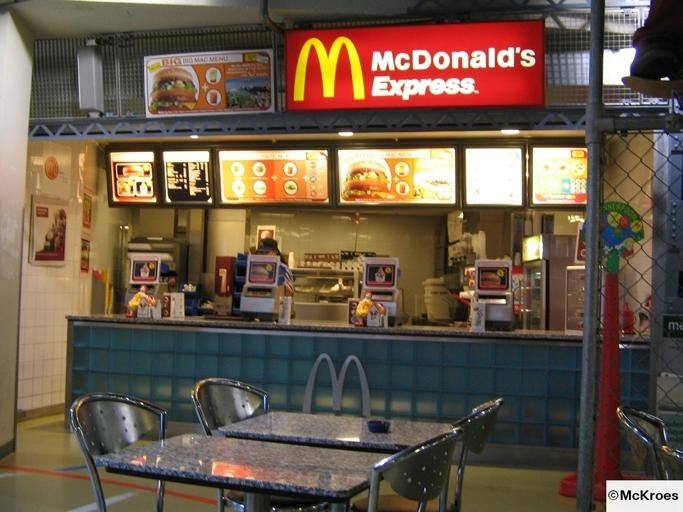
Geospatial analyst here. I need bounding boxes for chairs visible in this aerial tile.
[68,378,506,512]
[615,403,683,480]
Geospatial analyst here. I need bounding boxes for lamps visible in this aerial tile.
[76,39,105,118]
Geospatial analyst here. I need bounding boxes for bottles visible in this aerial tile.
[622,301,635,336]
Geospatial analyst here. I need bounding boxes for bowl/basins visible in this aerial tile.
[366,421,390,434]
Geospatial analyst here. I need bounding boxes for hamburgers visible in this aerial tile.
[148,68,197,114]
[341,161,393,201]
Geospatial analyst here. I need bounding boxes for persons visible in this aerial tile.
[255,237,295,319]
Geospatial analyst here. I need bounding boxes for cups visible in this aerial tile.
[278,296,292,326]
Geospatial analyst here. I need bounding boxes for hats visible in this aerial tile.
[255,239,277,254]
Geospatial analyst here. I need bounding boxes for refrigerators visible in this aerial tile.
[522,233,576,331]
